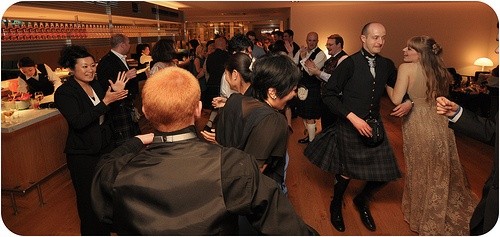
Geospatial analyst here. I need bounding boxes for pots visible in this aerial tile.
[13,100,30,110]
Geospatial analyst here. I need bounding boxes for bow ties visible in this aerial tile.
[120,55,127,61]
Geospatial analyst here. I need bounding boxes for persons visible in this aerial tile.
[436,22,499,236]
[182,29,348,197]
[135,39,178,78]
[89,66,311,236]
[301,22,414,233]
[97,33,138,139]
[54,46,129,236]
[387,35,479,236]
[17,56,63,104]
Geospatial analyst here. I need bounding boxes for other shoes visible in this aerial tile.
[203,125,215,133]
[297,136,309,144]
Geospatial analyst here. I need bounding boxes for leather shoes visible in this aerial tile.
[330,200,345,232]
[353,196,376,232]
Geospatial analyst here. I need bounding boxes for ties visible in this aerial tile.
[361,50,376,79]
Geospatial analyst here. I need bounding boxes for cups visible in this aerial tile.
[35,91,44,101]
[1,111,22,125]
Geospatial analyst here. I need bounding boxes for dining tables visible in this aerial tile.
[1,88,71,215]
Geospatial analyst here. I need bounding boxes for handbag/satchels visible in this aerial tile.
[359,118,384,148]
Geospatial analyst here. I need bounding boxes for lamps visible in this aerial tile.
[474,58,494,72]
